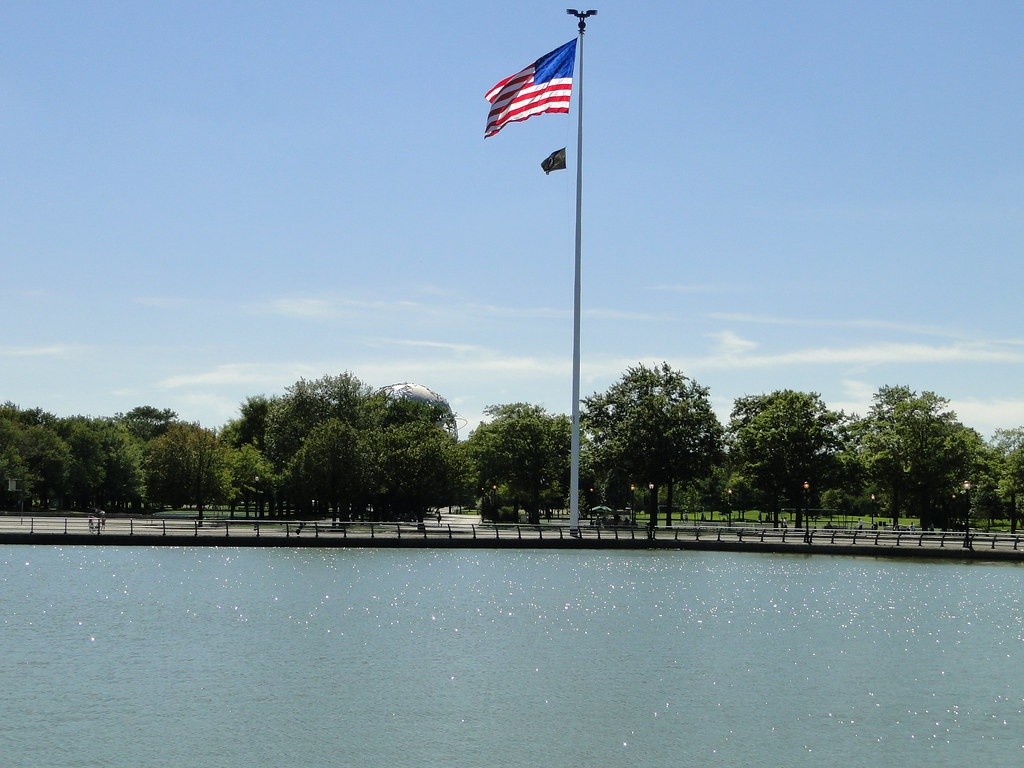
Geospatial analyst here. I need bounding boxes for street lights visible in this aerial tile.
[630,483,635,525]
[870,494,878,524]
[588,486,595,527]
[480,487,485,522]
[802,480,810,543]
[727,488,732,528]
[492,485,497,496]
[254,476,262,519]
[962,480,972,548]
[951,494,958,530]
[648,482,656,530]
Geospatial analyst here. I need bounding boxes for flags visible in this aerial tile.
[484,36,582,137]
[541,146,568,176]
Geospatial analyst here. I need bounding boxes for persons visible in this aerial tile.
[102,517,106,529]
[436,513,442,526]
[780,517,787,531]
[684,513,688,523]
[910,522,915,535]
[701,513,704,521]
[874,522,878,534]
[596,514,600,524]
[765,514,771,521]
[929,521,936,535]
[857,518,863,533]
[827,522,831,529]
[623,517,630,525]
[758,513,762,522]
[894,521,899,531]
